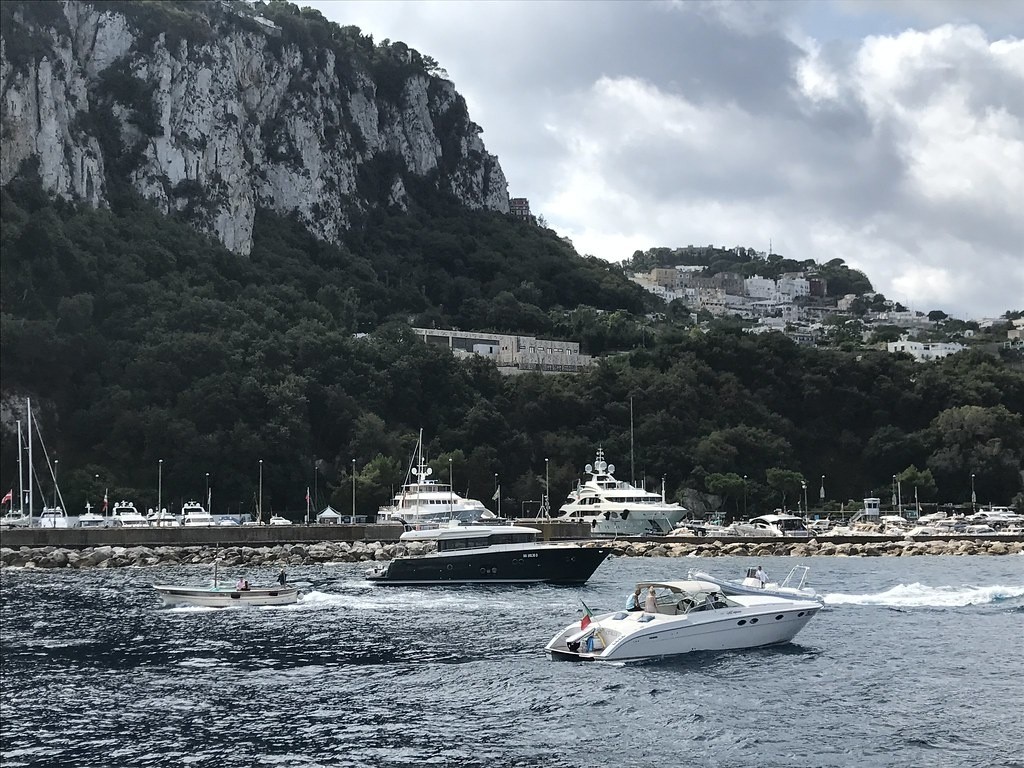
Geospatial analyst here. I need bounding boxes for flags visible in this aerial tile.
[492,487,499,500]
[102,495,108,511]
[581,605,591,631]
[1,490,11,504]
[207,491,211,504]
[579,601,593,620]
[305,490,309,504]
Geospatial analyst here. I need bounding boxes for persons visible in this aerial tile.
[276,569,288,588]
[755,566,770,589]
[644,586,658,613]
[236,576,248,591]
[626,586,644,612]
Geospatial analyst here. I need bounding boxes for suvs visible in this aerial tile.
[270,516,292,525]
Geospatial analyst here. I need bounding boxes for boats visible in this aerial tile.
[27,396,108,528]
[364,519,614,584]
[556,445,686,535]
[376,427,507,522]
[0,420,32,527]
[721,514,810,536]
[147,508,180,527]
[111,501,147,527]
[687,564,824,608]
[545,564,823,663]
[810,512,1024,535]
[180,499,214,526]
